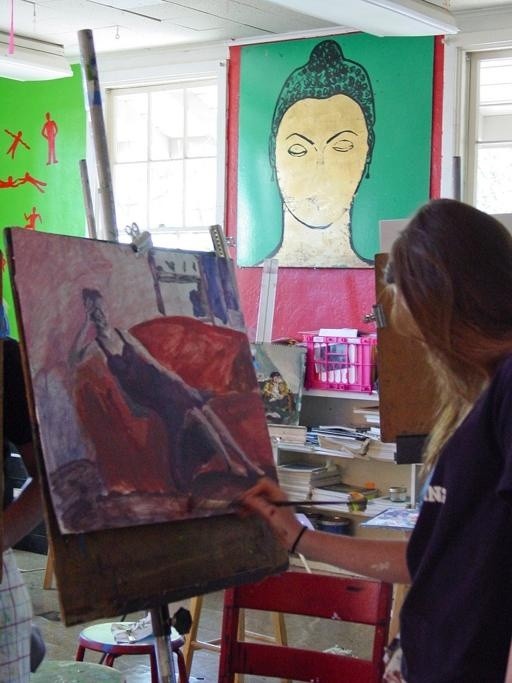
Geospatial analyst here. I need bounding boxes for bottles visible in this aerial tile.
[388,486,407,502]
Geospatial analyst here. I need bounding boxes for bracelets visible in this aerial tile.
[288,525,311,554]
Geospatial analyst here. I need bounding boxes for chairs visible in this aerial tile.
[217,573,393,681]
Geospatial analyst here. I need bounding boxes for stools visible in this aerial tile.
[78,622,190,682]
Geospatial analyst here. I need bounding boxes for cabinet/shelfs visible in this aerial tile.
[268,383,420,536]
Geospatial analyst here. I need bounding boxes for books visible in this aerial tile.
[263,398,399,504]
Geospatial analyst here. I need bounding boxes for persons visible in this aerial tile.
[0,336,47,683]
[65,280,271,480]
[220,200,511,683]
[253,39,381,266]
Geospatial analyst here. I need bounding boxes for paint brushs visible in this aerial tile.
[187,498,375,509]
